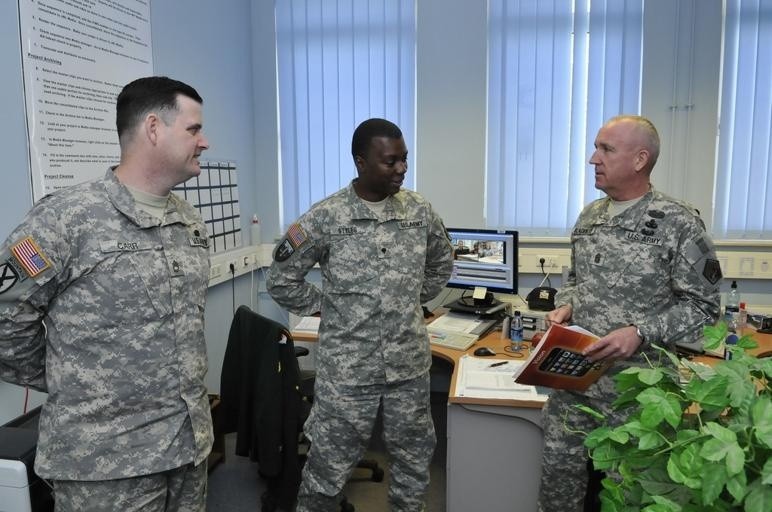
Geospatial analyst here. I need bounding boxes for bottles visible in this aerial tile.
[510,311,523,352]
[250,214,261,245]
[725,280,746,329]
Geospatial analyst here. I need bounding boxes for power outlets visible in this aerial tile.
[535,254,558,267]
[227,258,238,273]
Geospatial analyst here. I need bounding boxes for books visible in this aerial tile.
[512,323,618,394]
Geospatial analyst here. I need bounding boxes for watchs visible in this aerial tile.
[635,327,644,338]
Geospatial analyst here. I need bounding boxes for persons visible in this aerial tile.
[0,74,216,512]
[537,113,725,511]
[266,117,455,511]
[453,239,498,260]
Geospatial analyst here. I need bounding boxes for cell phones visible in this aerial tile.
[537,346,601,379]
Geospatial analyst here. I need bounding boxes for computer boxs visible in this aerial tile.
[444,299,512,324]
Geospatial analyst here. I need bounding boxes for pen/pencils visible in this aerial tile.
[490,361,509,366]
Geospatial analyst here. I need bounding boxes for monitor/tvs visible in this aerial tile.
[445,227,519,308]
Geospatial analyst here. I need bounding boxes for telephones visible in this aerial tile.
[526,287,558,310]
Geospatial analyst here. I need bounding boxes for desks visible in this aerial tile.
[289,306,554,512]
[675,323,772,418]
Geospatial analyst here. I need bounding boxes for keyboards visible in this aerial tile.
[427,326,480,351]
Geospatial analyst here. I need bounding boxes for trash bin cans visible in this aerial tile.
[206,394,225,476]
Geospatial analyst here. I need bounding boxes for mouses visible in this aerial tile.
[474,347,496,356]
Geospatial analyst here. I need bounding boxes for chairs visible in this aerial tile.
[234,302,384,512]
